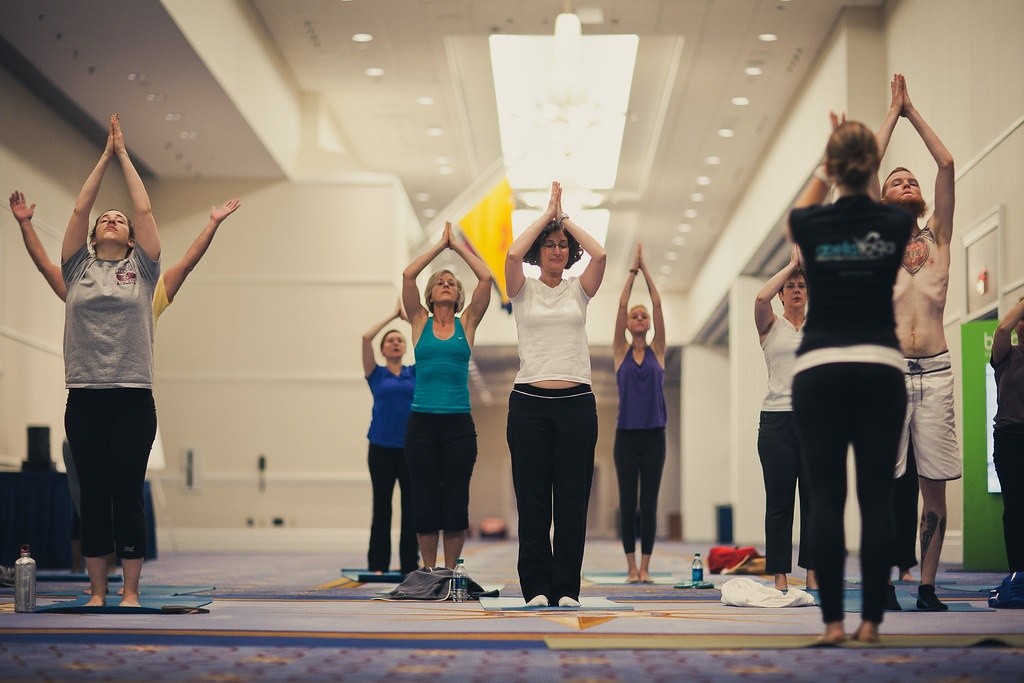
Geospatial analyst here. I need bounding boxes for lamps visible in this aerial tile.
[554,0,583,52]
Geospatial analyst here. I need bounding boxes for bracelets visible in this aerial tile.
[630,269,638,274]
[557,214,569,225]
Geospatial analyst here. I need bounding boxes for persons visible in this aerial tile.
[504,181,607,607]
[61,112,161,607]
[9,190,240,595]
[990,298,1024,577]
[402,221,493,567]
[755,244,818,590]
[613,242,665,584]
[362,297,420,574]
[784,112,914,644]
[896,427,918,581]
[867,74,963,609]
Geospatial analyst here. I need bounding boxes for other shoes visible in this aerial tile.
[883,585,901,611]
[916,585,949,611]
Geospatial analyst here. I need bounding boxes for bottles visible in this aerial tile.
[15,544,37,613]
[691,553,703,586]
[453,559,468,602]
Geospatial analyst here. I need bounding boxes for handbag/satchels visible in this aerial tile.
[707,546,757,573]
[989,571,1024,607]
[373,566,500,601]
[720,554,774,575]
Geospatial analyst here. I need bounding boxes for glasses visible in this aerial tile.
[541,239,570,248]
